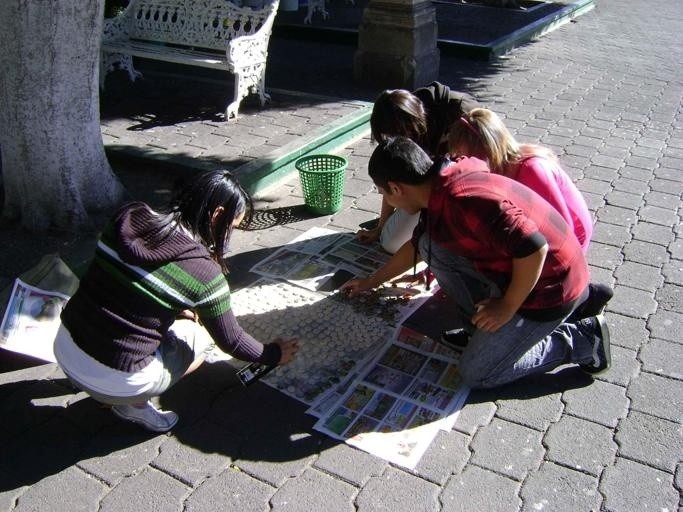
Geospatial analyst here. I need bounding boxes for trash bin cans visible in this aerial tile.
[295,154,348,215]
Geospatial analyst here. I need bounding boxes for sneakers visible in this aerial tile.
[113,400,178,433]
[440,327,472,352]
[577,283,613,376]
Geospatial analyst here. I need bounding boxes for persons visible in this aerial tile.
[353,81,593,256]
[53,169,299,432]
[339,137,610,390]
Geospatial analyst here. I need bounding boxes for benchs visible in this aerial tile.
[99,0,282,122]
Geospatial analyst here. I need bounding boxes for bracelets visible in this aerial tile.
[368,273,380,287]
